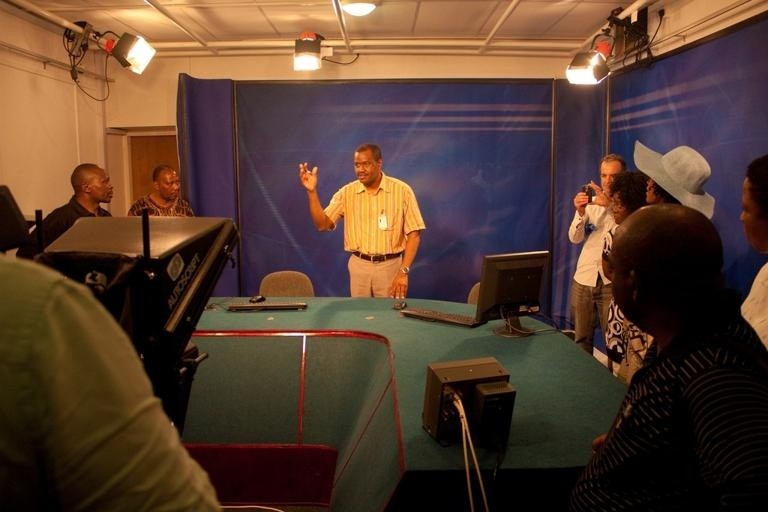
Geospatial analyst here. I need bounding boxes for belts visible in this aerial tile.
[353,252,403,263]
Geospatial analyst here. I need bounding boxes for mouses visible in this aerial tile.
[393,301,406,310]
[250,295,265,302]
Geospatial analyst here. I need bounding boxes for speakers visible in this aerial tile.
[422,357,516,452]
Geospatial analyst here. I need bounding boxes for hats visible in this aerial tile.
[633,140,715,219]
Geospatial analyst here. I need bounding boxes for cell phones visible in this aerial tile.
[582,185,593,203]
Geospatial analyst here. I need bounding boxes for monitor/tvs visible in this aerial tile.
[477,250,552,339]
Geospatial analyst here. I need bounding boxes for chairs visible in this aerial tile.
[467,282,482,304]
[259,271,316,297]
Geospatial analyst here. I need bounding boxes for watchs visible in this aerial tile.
[400,266,410,274]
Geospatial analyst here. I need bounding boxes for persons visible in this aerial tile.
[18,164,114,258]
[127,165,194,217]
[567,154,627,354]
[299,143,427,300]
[571,202,766,510]
[0,188,220,512]
[602,171,647,374]
[740,155,768,352]
[618,140,715,382]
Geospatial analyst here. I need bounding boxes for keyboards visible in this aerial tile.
[228,302,306,311]
[400,307,476,329]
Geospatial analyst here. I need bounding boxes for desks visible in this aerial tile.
[178,297,631,511]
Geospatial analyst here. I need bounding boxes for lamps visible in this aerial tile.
[337,0,381,16]
[294,35,322,72]
[112,33,157,74]
[566,50,611,86]
